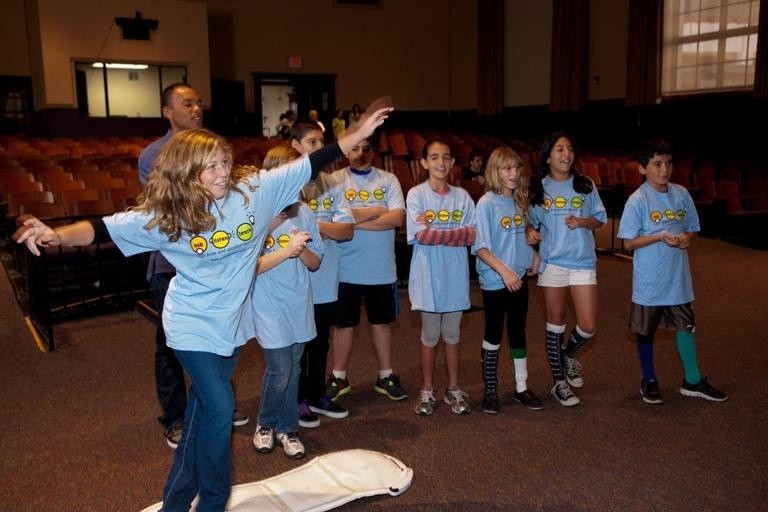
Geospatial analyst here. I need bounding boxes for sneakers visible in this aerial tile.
[640,378,664,404]
[679,375,728,402]
[253,421,275,454]
[550,378,581,407]
[305,394,349,419]
[325,372,352,402]
[443,386,472,415]
[274,431,306,459]
[415,388,437,416]
[560,344,584,388]
[513,389,544,410]
[480,392,499,414]
[164,418,184,449]
[297,402,321,428]
[233,409,250,426]
[375,373,409,400]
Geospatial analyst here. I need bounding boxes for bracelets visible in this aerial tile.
[659,232,664,241]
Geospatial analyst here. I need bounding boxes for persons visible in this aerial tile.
[253,103,406,459]
[406,135,476,415]
[619,142,727,403]
[466,146,544,416]
[136,81,249,450]
[526,135,606,407]
[18,107,398,511]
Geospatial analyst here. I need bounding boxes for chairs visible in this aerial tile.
[317,112,768,261]
[0,134,287,350]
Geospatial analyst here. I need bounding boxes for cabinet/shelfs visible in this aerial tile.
[0,75,36,130]
